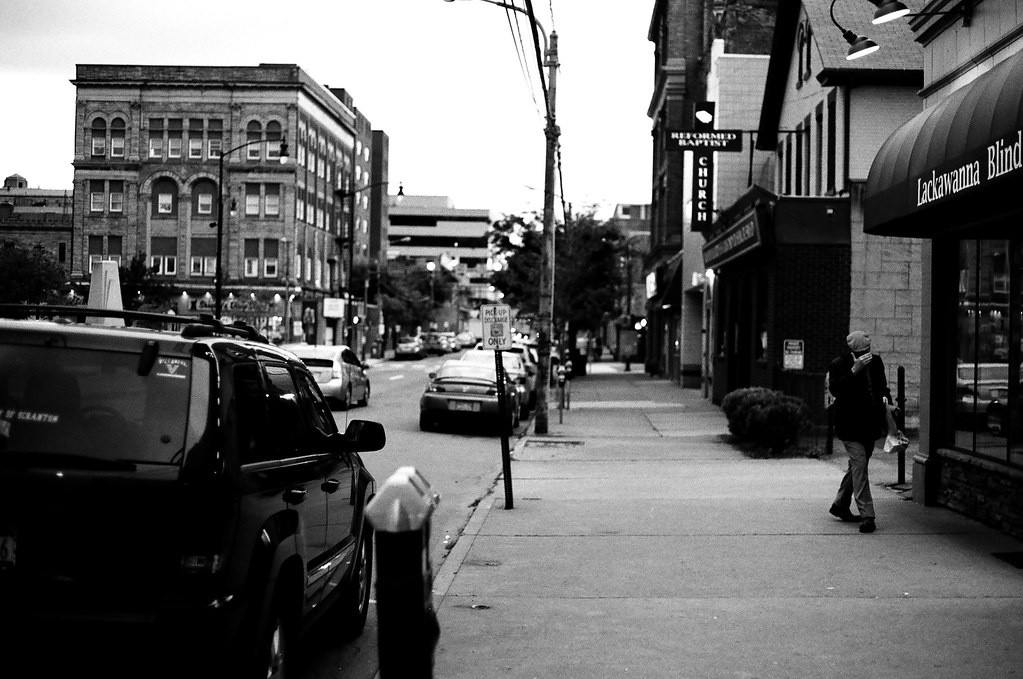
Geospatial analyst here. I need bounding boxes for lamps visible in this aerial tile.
[829,0,975,61]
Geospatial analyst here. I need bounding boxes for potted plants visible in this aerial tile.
[718,385,810,458]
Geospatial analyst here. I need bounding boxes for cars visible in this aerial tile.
[270,343,370,410]
[419,360,525,436]
[396,336,424,358]
[442,333,461,352]
[457,333,475,346]
[476,340,562,393]
[958,363,1008,413]
[460,350,530,417]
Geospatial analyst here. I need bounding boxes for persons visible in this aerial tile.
[827,333,895,535]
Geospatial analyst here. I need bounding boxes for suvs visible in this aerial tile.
[0,302,386,679]
[418,332,447,356]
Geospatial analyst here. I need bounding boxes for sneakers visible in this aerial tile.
[859,517,876,533]
[829,503,860,521]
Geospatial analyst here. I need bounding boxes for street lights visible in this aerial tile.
[445,0,561,433]
[348,180,405,348]
[216,137,289,324]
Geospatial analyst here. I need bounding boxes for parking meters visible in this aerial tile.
[557,364,566,424]
[565,360,574,411]
[362,464,440,679]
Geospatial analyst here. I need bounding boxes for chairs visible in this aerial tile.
[141,370,185,434]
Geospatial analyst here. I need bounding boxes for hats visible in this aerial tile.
[846,330,871,353]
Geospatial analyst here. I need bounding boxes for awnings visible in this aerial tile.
[836,69,1023,234]
